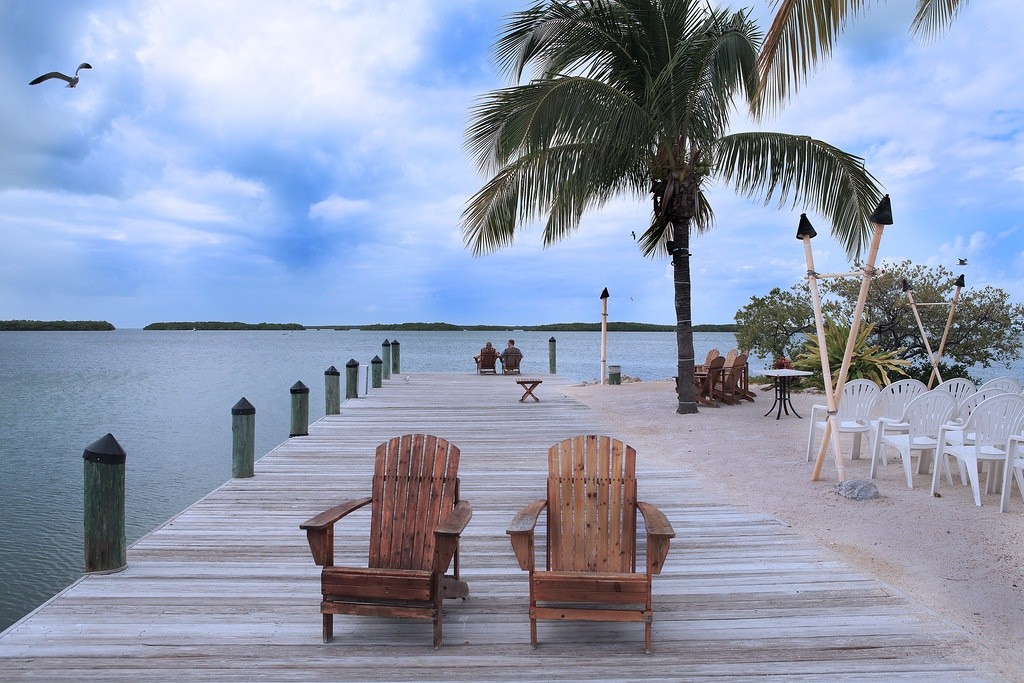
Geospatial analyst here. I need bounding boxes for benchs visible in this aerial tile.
[516,378,543,402]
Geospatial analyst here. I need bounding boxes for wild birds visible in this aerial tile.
[29,63,92,88]
[956,258,968,265]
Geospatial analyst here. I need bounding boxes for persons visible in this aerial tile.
[472,342,498,364]
[500,340,524,363]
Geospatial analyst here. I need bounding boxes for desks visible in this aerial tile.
[753,368,813,419]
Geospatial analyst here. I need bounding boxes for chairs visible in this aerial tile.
[502,347,521,374]
[672,349,757,408]
[298,433,473,650]
[805,376,1024,513]
[506,434,677,654]
[472,347,496,375]
